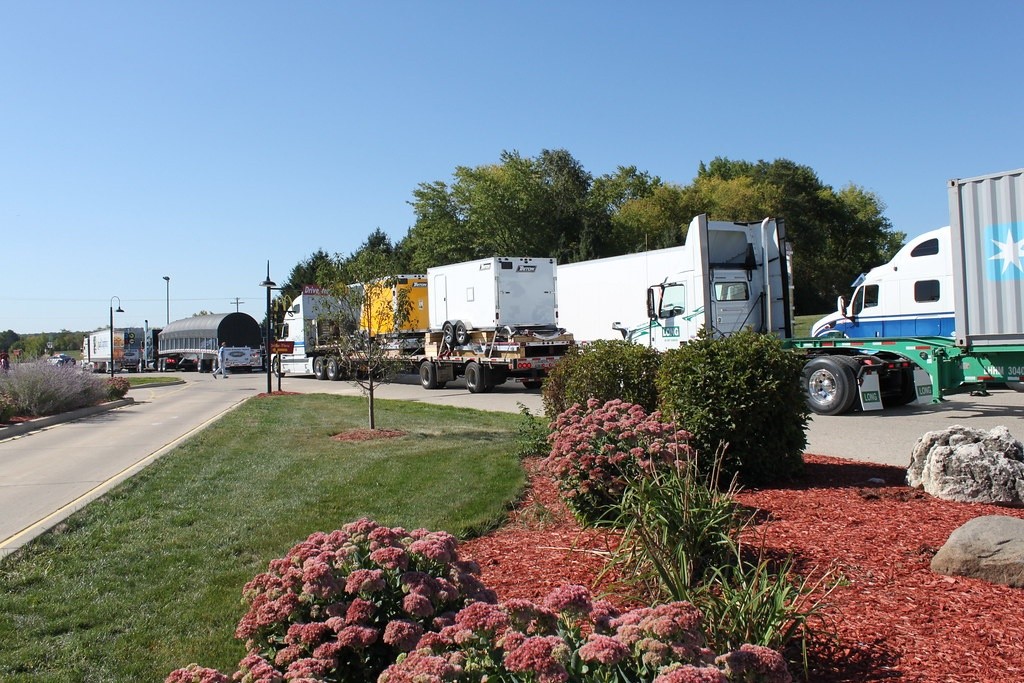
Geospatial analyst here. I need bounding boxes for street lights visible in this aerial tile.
[110,296,125,377]
[257,259,277,392]
[162,276,170,325]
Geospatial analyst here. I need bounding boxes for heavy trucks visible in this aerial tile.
[612,167,1024,414]
[82,328,144,373]
[139,312,263,375]
[272,288,575,395]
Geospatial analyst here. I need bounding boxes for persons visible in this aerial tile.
[212,342,228,378]
[0,350,10,373]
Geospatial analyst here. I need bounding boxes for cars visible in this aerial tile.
[49,355,76,367]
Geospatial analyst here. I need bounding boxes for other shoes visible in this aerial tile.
[223,376,228,378]
[213,373,217,379]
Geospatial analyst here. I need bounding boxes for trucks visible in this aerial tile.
[811,224,955,338]
